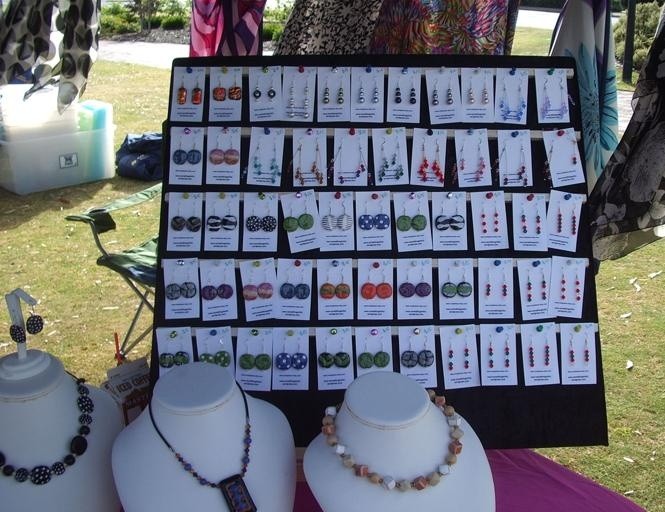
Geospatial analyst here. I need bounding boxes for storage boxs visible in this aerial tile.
[0,84,116,194]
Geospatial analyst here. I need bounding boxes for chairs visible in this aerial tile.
[65,182,163,356]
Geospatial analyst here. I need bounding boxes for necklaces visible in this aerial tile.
[1,372,94,485]
[320,391,463,493]
[141,381,257,511]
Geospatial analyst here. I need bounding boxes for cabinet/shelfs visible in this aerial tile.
[298,438,641,510]
